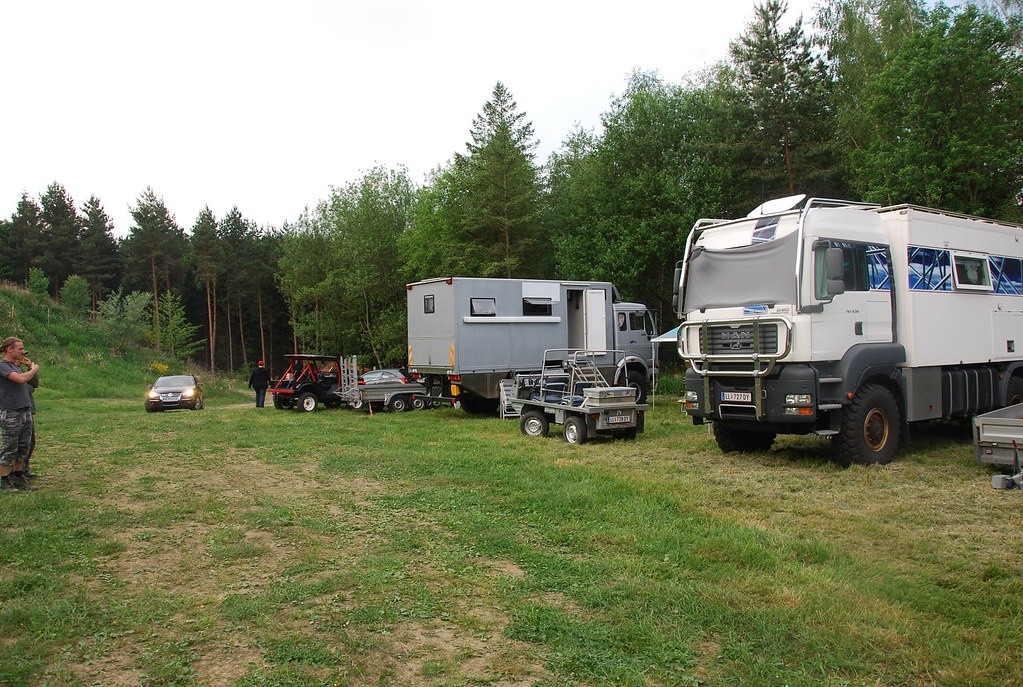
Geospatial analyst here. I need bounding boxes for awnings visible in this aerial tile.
[472,299,496,314]
[524,298,557,305]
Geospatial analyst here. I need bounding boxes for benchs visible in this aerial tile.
[532,377,591,406]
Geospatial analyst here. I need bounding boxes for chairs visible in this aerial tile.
[499,379,520,419]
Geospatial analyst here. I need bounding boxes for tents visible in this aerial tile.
[650,326,685,411]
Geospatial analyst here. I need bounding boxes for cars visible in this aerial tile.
[360,369,417,384]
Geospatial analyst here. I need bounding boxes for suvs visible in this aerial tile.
[145,374,203,412]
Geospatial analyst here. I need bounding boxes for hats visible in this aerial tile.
[258,360,264,365]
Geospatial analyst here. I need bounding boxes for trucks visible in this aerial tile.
[673,194,1023,468]
[333,355,442,412]
[406,277,659,414]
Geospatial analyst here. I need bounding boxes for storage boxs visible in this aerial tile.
[583,387,637,403]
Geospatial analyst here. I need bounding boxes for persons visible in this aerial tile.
[0,336,39,491]
[248,361,272,408]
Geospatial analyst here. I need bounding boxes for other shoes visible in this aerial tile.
[9,474,30,481]
[0,476,19,492]
[12,475,38,491]
[27,472,37,478]
